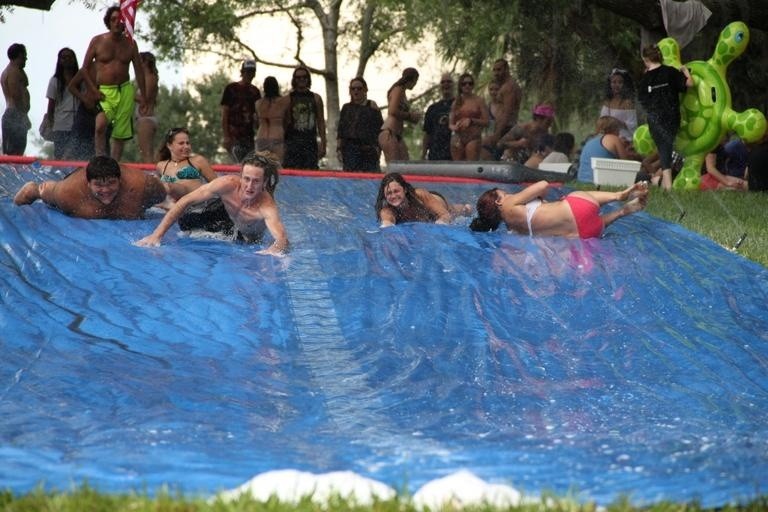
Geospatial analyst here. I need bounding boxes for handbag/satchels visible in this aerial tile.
[40,114,54,141]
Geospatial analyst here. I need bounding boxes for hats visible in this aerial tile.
[242,60,256,71]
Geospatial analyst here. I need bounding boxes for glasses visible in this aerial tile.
[169,128,177,131]
[245,154,268,164]
[459,80,473,86]
[350,87,362,91]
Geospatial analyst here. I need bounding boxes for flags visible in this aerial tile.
[121,0,139,43]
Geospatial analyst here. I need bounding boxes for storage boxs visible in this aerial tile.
[590,157,642,188]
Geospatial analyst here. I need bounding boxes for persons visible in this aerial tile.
[47,8,161,163]
[336,68,421,172]
[129,152,291,256]
[11,154,166,221]
[151,131,219,214]
[466,180,654,238]
[375,171,474,226]
[1,43,33,159]
[219,60,326,170]
[576,44,693,191]
[702,129,766,192]
[421,59,576,179]
